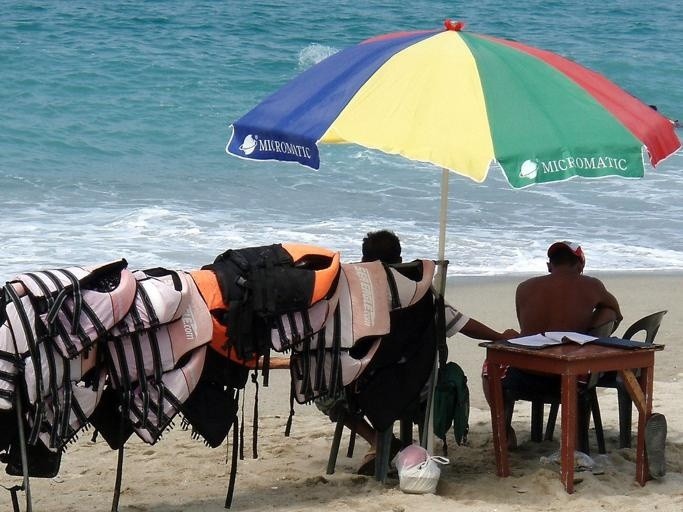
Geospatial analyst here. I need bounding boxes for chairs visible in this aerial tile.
[326,259,450,480]
[543,307,667,457]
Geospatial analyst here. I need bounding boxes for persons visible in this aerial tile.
[479,241,624,451]
[315,229,522,477]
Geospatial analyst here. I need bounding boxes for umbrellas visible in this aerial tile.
[225,19,681,458]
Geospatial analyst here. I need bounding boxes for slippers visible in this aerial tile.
[358,437,402,476]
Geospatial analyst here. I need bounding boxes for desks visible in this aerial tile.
[479,330,665,496]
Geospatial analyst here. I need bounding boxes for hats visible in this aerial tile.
[547,240,585,272]
